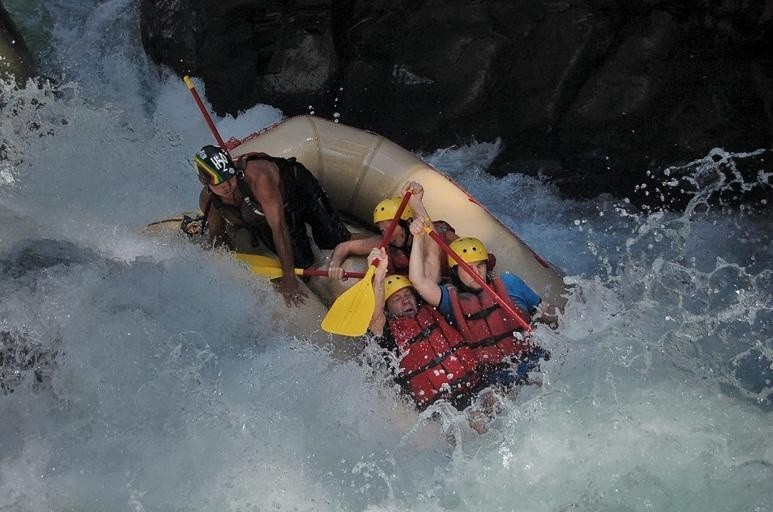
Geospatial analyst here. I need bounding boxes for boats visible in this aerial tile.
[207,114,569,451]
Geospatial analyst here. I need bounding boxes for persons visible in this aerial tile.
[196,144,369,310]
[408,217,549,390]
[366,182,500,459]
[327,196,461,283]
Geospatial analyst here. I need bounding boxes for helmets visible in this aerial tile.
[382,274,415,307]
[191,144,237,186]
[446,236,490,269]
[372,195,415,225]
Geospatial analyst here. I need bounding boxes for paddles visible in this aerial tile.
[321,187,413,337]
[230,252,375,285]
